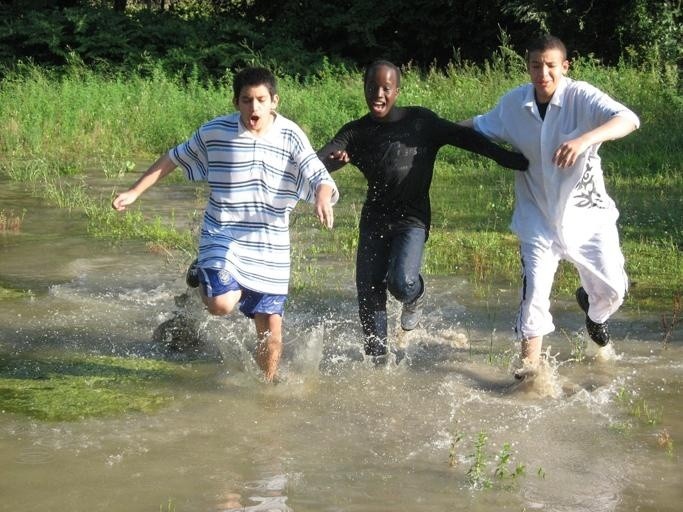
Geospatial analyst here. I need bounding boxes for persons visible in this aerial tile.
[112,67,340,387]
[327,34,640,384]
[313,59,529,374]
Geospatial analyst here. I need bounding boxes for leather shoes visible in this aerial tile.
[572,284,613,348]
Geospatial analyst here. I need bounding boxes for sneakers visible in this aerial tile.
[184,255,203,291]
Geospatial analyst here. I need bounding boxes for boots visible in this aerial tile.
[397,271,428,332]
